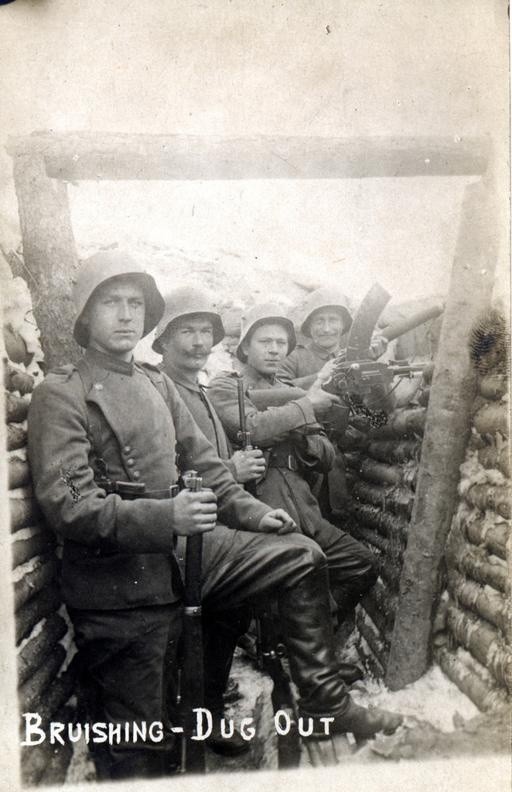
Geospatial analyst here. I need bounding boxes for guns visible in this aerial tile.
[175,470,205,773]
[234,371,303,767]
[247,281,435,412]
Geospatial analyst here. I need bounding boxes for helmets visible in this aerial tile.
[152,287,225,355]
[300,288,353,336]
[235,304,297,363]
[73,249,166,348]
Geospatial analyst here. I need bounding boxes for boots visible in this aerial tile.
[202,629,252,757]
[241,571,404,741]
[109,753,166,779]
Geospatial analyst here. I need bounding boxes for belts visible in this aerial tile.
[270,454,300,471]
[140,483,181,500]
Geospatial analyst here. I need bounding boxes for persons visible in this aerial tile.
[27,249,405,783]
[206,302,382,702]
[151,286,266,773]
[238,287,388,658]
